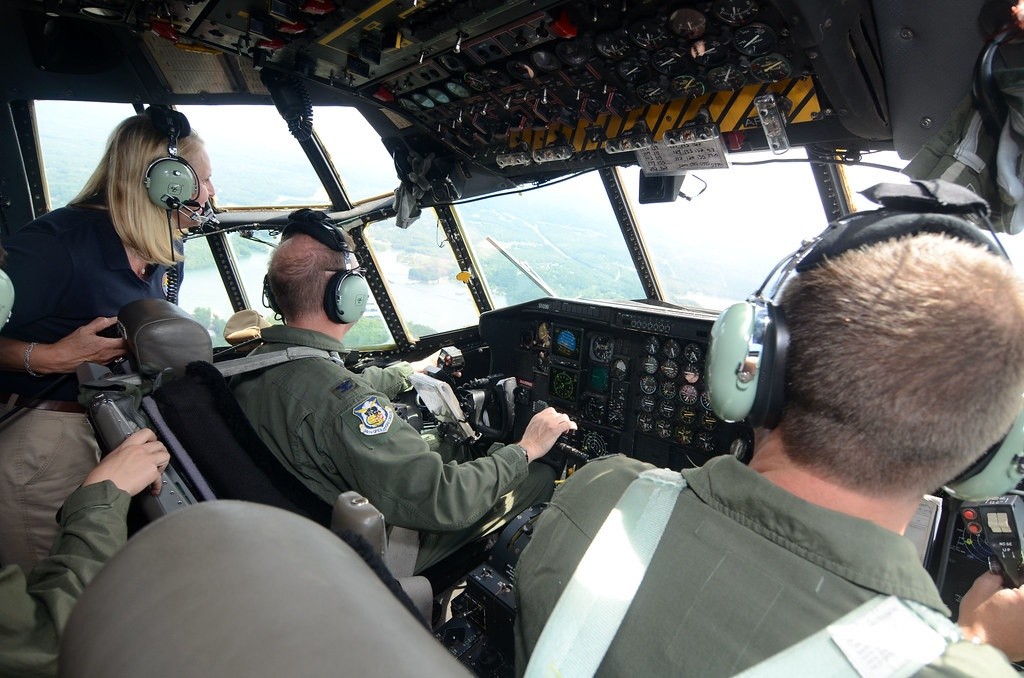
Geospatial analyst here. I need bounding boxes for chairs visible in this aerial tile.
[55,499,475,678]
[115,298,333,529]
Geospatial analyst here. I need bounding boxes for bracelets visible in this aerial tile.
[23,342,45,377]
[518,445,528,464]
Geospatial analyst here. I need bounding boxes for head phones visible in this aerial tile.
[263,208,367,324]
[144,106,201,211]
[0,267,15,332]
[703,170,1022,500]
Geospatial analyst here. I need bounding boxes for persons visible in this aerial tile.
[0,113,215,402]
[0,428,171,678]
[228,208,556,578]
[514,204,1024,678]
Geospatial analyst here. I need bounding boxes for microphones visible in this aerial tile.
[161,194,214,224]
